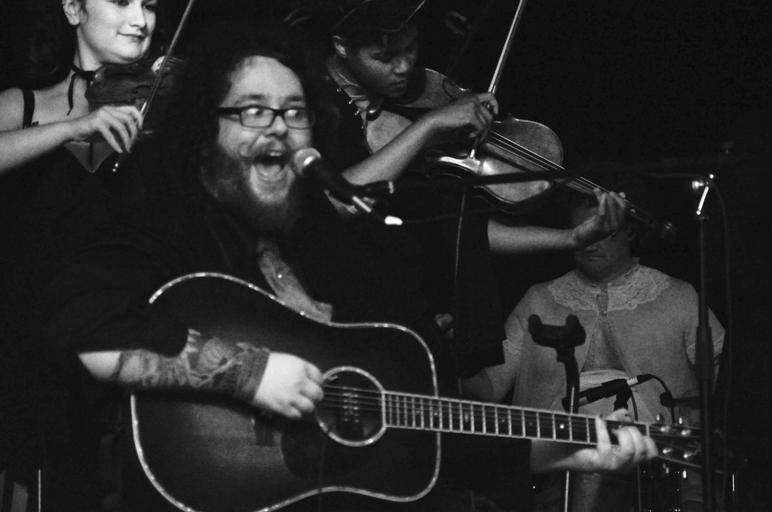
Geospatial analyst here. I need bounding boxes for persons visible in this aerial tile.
[72,36,659,512]
[471,199,726,512]
[298,0,629,339]
[0,0,155,323]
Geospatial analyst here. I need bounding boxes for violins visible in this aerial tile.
[86,48,214,104]
[363,68,681,238]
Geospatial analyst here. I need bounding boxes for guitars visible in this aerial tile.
[113,271,726,511]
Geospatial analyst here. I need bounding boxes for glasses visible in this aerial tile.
[212,103,316,132]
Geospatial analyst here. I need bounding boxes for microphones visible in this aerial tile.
[293,147,374,216]
[562,374,652,412]
